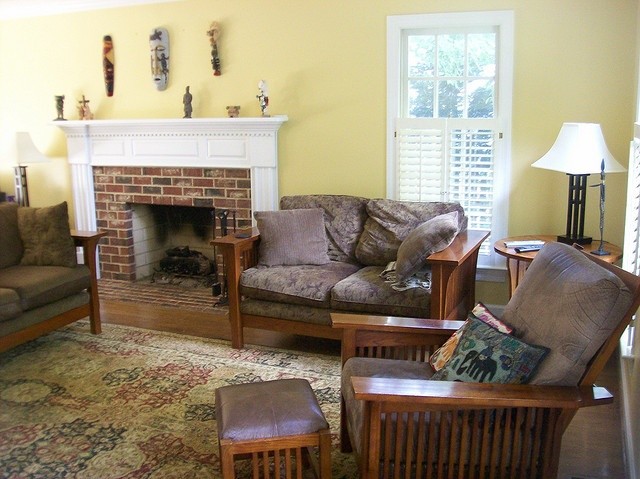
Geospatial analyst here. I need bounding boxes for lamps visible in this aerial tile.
[532,123,628,244]
[0,131,47,206]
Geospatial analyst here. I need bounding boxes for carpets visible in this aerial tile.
[0,322,360,477]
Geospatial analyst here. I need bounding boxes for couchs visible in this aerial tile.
[329,242,640,478]
[0,200,107,352]
[210,195,491,350]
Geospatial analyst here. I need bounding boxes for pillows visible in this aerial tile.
[17,202,77,268]
[428,302,522,371]
[427,310,549,430]
[396,211,459,284]
[254,207,330,264]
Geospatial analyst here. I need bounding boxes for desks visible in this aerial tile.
[494,234,622,302]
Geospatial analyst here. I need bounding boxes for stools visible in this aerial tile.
[215,379,332,478]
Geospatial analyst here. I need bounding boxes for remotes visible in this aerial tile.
[515,245,544,252]
[504,240,546,247]
